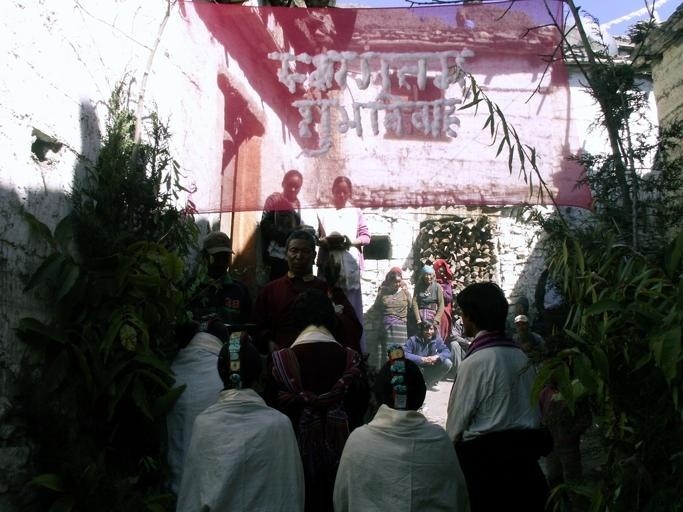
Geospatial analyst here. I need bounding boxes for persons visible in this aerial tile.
[331,355,474,512]
[172,330,307,512]
[313,175,371,361]
[444,280,551,511]
[251,230,364,354]
[162,309,239,495]
[193,230,253,340]
[262,322,371,511]
[257,169,303,281]
[455,7,475,30]
[372,257,551,395]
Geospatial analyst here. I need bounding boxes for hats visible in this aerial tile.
[514,315,530,324]
[516,297,529,306]
[202,231,234,255]
